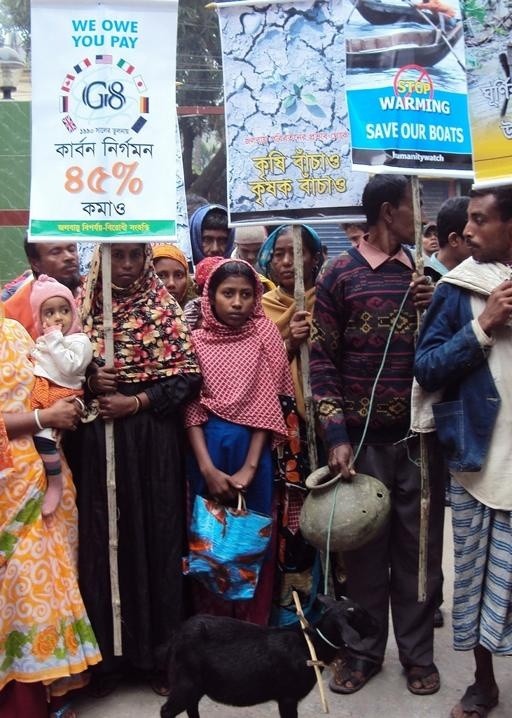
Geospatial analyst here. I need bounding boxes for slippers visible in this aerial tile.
[405,662,441,694]
[451,684,499,717]
[48,699,76,718]
[329,657,382,693]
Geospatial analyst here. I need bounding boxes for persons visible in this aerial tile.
[133,188,478,343]
[58,239,205,690]
[253,221,324,595]
[409,184,512,718]
[2,234,86,344]
[1,300,107,718]
[181,256,297,628]
[29,273,93,518]
[307,170,443,698]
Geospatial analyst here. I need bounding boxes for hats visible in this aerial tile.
[29,273,77,336]
[422,221,437,234]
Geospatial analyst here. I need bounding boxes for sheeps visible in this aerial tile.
[160,594,383,718]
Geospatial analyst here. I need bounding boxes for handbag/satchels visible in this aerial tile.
[181,490,274,602]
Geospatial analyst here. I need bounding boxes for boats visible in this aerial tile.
[349,0,458,26]
[346,21,462,71]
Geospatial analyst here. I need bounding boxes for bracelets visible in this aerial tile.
[35,409,44,431]
[130,395,140,416]
[75,398,85,412]
